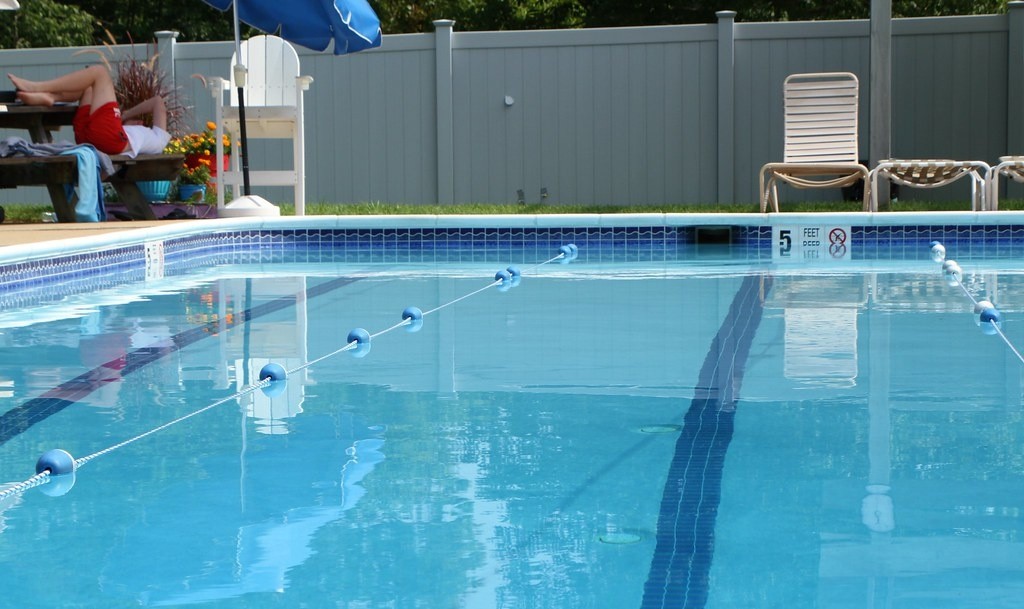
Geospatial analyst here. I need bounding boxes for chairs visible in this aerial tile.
[218,280,307,435]
[211,34,313,216]
[758,71,871,213]
[757,272,868,388]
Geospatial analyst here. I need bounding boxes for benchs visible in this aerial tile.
[0,154,185,224]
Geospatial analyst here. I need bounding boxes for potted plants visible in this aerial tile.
[73,29,205,204]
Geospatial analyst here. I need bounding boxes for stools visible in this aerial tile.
[871,270,1024,314]
[986,156,1024,212]
[872,158,991,211]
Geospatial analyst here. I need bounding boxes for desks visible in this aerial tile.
[0,105,78,223]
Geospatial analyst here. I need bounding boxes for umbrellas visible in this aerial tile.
[203,0,382,196]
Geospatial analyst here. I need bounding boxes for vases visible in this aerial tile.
[186,152,228,178]
[178,185,206,202]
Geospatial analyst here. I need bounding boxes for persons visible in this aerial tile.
[9,65,172,158]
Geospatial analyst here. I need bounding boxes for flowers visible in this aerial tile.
[164,121,241,181]
[181,286,236,337]
